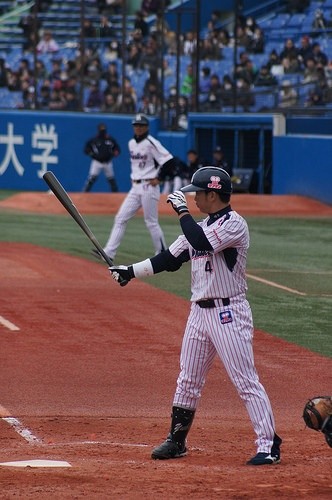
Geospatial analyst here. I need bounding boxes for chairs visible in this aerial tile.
[0,1,331,117]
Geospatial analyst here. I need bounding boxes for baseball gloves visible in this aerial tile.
[303,396,332,448]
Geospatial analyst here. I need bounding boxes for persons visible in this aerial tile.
[161,147,233,196]
[83,122,122,192]
[89,113,178,266]
[0,1,332,122]
[107,167,282,468]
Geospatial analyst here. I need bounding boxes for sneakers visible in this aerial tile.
[179,166,233,193]
[246,452,281,465]
[89,249,113,265]
[151,440,188,459]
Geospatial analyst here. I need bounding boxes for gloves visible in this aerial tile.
[167,191,189,215]
[108,264,135,286]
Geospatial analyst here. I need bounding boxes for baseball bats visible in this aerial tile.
[44,171,127,287]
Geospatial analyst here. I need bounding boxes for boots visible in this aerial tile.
[108,176,118,192]
[84,176,97,192]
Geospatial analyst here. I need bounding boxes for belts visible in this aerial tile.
[196,297,230,308]
[134,180,147,183]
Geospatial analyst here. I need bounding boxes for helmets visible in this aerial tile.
[132,114,149,125]
